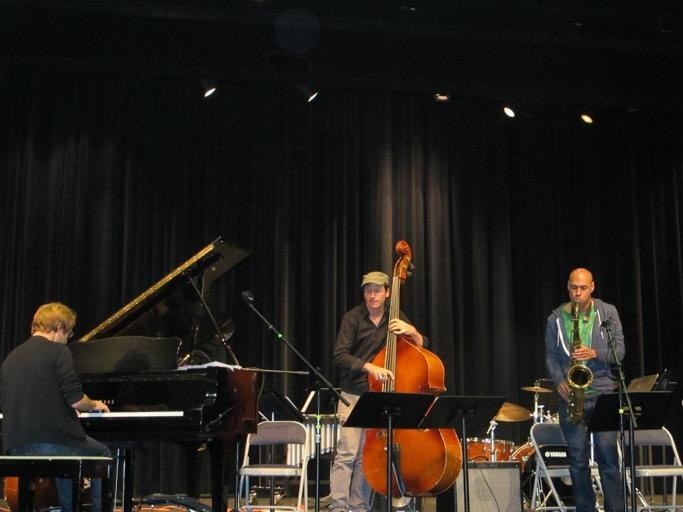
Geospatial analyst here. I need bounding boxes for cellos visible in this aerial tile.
[362,243,464,499]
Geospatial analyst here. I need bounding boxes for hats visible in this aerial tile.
[360,271,389,287]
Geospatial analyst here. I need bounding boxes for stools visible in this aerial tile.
[0,456,113,511]
[436,462,524,512]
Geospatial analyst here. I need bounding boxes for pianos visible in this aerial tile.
[67,232,256,512]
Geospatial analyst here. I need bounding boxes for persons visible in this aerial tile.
[327,271,429,512]
[0,302,113,512]
[545,268,628,512]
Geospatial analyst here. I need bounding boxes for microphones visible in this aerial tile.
[240,290,255,306]
[601,318,614,330]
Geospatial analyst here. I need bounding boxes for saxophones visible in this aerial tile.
[566,301,593,427]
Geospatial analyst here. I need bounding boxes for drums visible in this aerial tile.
[511,443,580,510]
[460,438,515,463]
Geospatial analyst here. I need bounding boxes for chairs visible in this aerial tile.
[530,422,604,511]
[238,421,308,512]
[617,426,683,512]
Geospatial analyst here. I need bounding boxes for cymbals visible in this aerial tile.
[495,402,534,423]
[521,386,552,392]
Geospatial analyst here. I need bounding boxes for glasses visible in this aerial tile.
[68,329,74,339]
